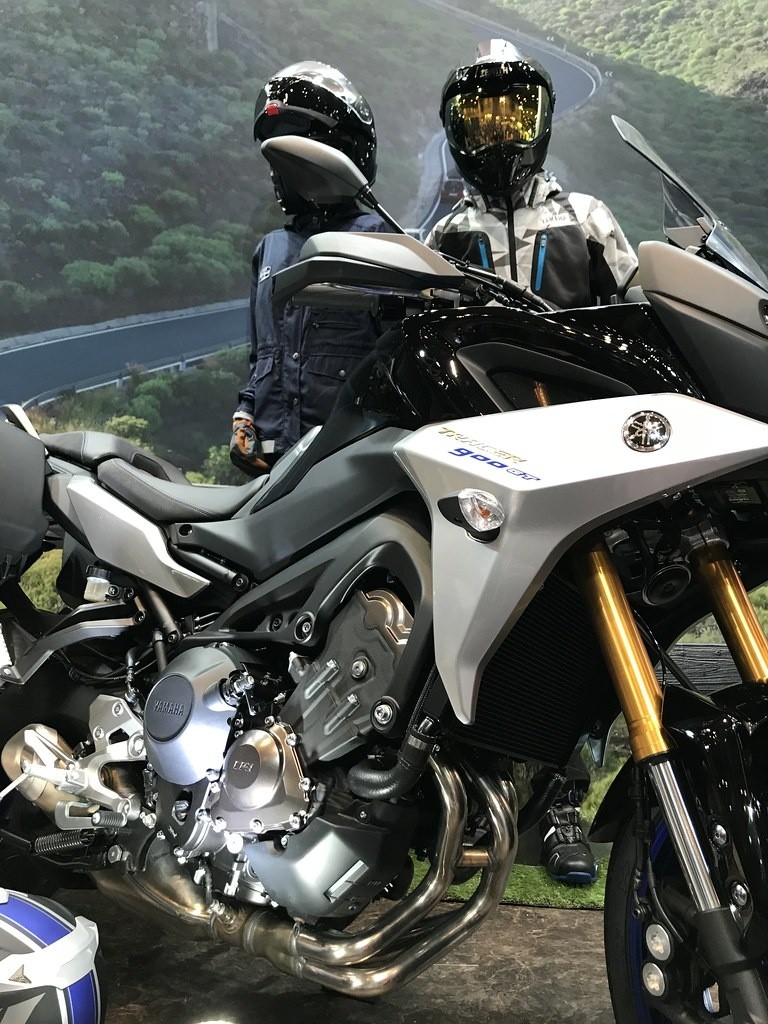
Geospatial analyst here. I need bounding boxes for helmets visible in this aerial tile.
[252,61,377,212]
[440,37,555,194]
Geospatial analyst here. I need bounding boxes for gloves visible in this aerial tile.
[229,417,270,476]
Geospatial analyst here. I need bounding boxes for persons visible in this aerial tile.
[424,38,647,883]
[229,58,446,480]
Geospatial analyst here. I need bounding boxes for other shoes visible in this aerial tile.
[543,808,599,885]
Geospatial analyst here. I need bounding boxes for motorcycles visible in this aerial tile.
[0,114,768,1021]
